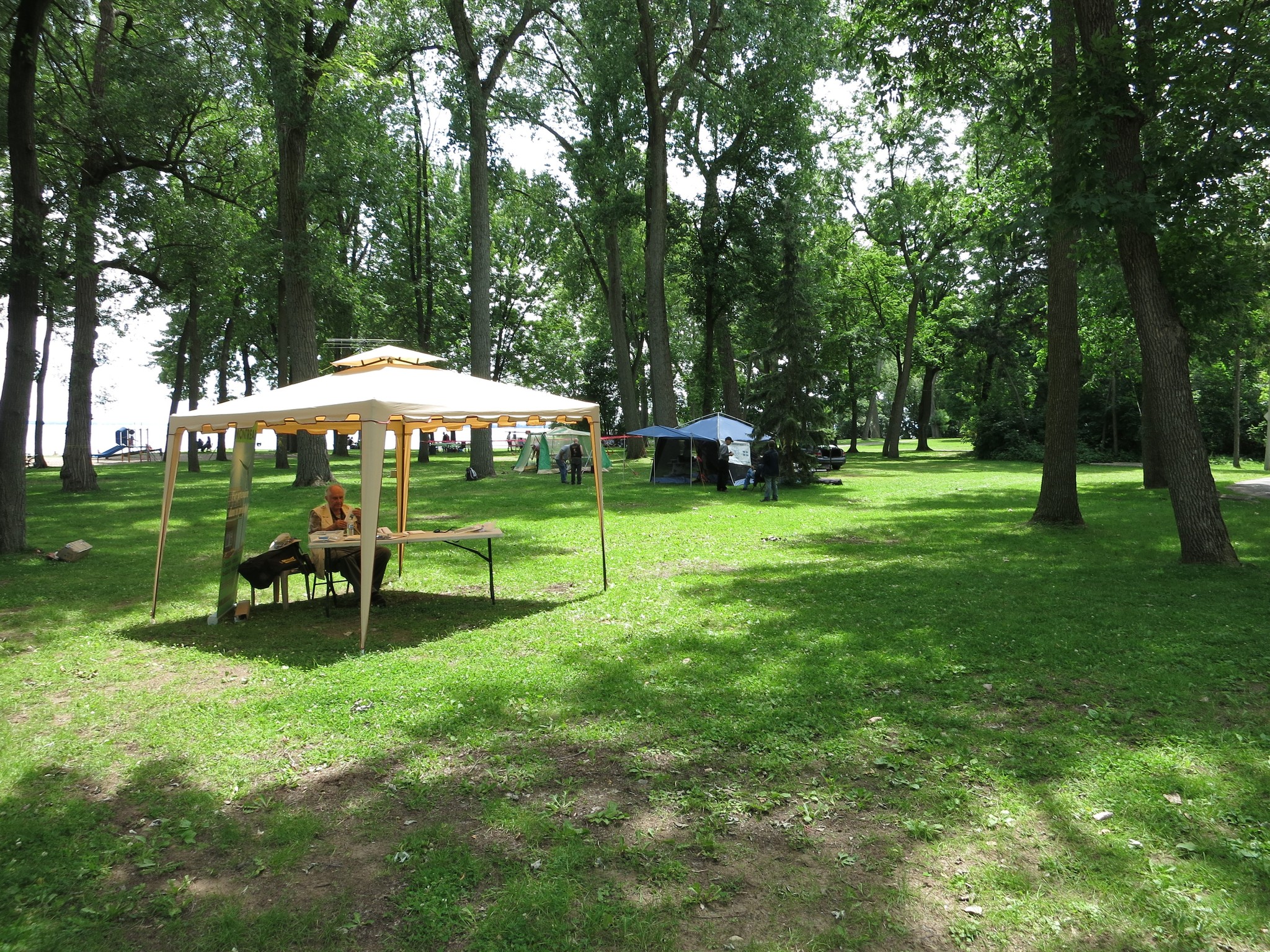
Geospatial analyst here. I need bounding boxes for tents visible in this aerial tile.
[512,426,613,475]
[626,425,720,494]
[673,412,780,492]
[144,343,610,658]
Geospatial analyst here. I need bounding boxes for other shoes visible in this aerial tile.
[562,481,569,484]
[370,592,388,607]
[760,498,770,501]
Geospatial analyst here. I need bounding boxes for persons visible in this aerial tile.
[441,432,448,453]
[347,437,350,443]
[518,437,524,452]
[308,485,392,608]
[201,436,211,452]
[717,437,735,493]
[347,437,356,448]
[445,434,450,441]
[554,442,575,483]
[506,431,514,452]
[198,438,204,445]
[742,455,767,491]
[567,438,585,486]
[530,443,540,473]
[759,440,780,503]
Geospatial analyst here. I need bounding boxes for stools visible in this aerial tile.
[313,565,362,605]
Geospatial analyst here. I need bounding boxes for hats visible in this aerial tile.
[768,440,775,447]
[725,437,734,442]
[266,533,302,551]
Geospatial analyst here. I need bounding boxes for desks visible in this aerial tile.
[308,523,503,607]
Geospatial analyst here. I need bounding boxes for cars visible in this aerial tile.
[796,433,845,474]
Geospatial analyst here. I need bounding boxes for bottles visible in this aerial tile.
[346,518,354,536]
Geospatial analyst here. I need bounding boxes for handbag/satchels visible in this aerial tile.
[238,539,315,590]
[466,466,479,481]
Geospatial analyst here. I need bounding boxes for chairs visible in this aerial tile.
[269,534,333,610]
